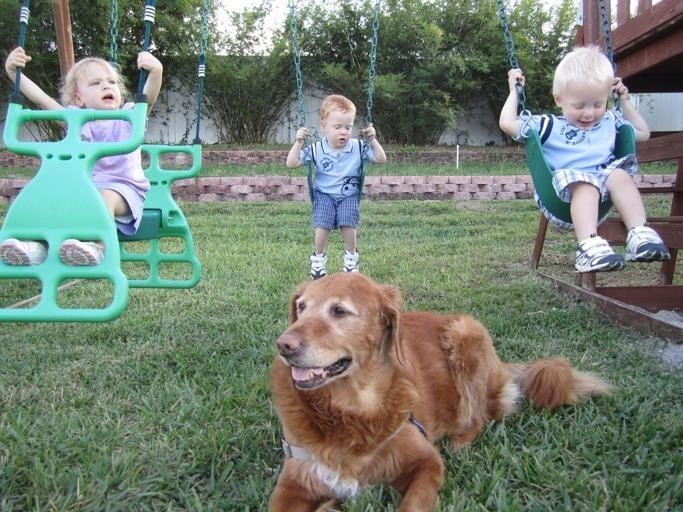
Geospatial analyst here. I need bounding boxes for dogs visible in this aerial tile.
[267,272,621,512]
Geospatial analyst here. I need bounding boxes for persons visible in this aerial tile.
[497,42,673,273]
[286,93,387,281]
[0,42,166,266]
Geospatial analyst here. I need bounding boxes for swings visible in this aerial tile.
[496,0,636,224]
[288,0,380,204]
[0,0,209,323]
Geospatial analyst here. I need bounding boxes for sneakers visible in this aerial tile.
[1,238,46,266]
[341,248,359,273]
[622,223,671,262]
[575,233,625,273]
[308,251,328,279]
[60,238,104,266]
